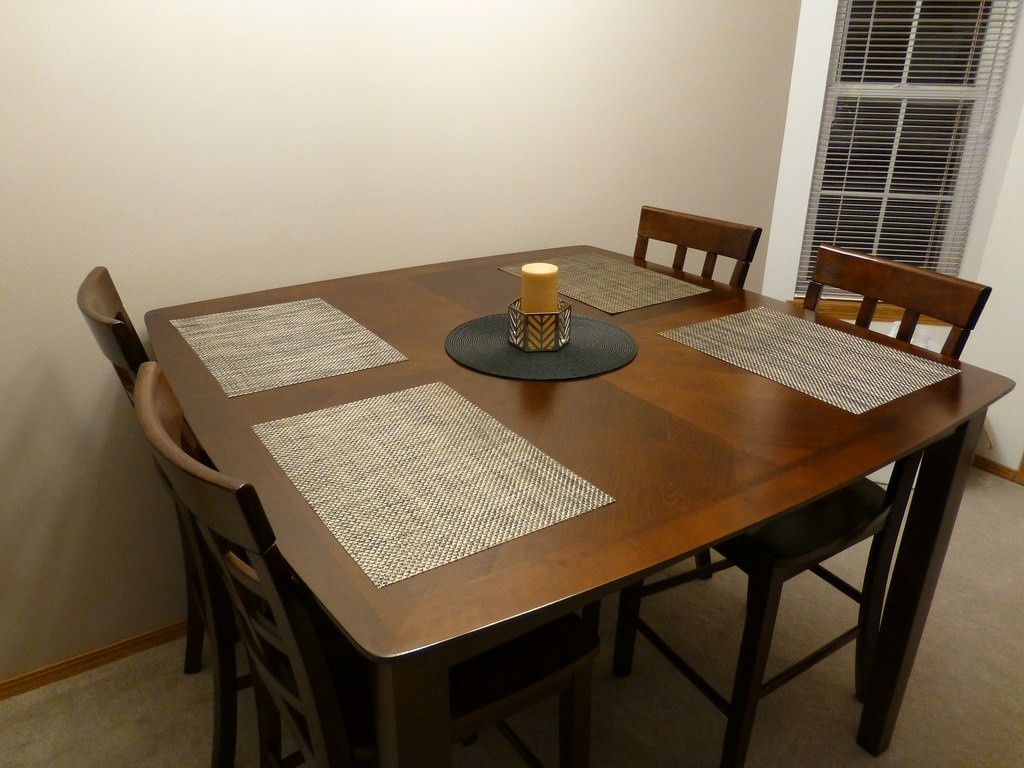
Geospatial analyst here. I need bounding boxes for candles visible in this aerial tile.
[522,263,559,348]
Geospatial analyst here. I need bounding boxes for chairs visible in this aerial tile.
[75,206,993,768]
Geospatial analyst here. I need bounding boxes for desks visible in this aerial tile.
[143,238,1017,768]
